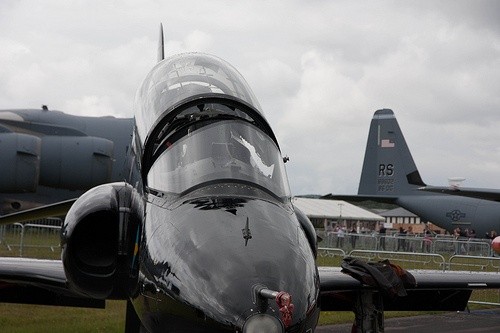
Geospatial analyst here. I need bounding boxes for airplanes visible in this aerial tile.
[0,22,165,225]
[292,108,500,239]
[1,51,500,332]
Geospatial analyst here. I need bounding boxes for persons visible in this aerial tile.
[349,226,359,248]
[379,224,386,250]
[396,224,497,257]
[336,224,345,249]
